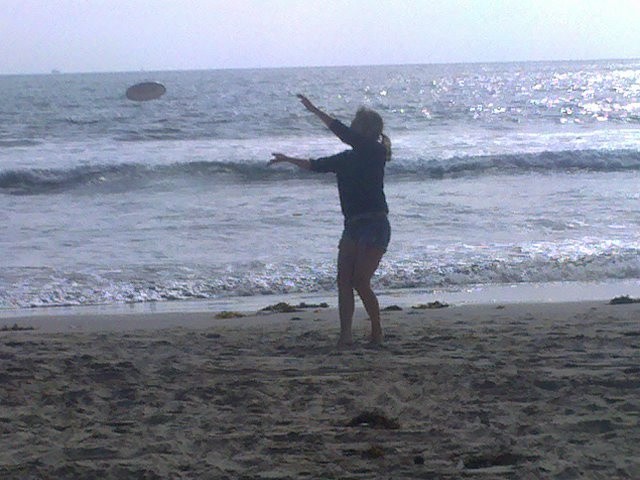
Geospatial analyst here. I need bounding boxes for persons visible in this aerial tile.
[266,93,390,350]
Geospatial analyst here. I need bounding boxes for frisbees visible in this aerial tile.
[126,81,167,101]
